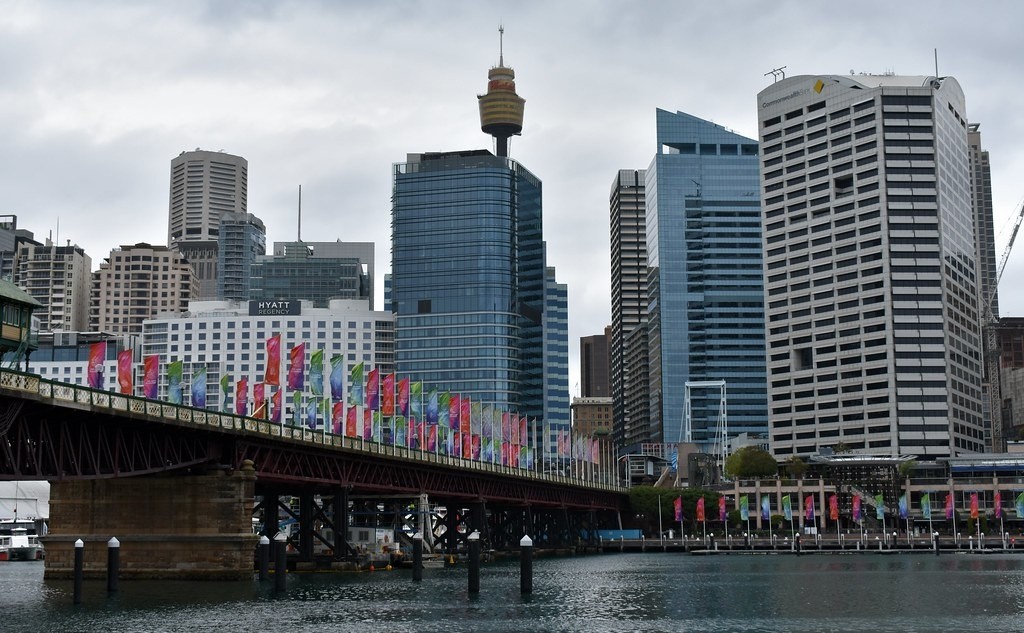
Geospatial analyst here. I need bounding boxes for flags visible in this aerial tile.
[875,494,884,520]
[829,495,838,520]
[1016,491,1024,519]
[761,495,770,521]
[921,493,930,519]
[994,492,1001,519]
[970,493,978,519]
[719,496,726,521]
[945,493,953,520]
[739,496,748,521]
[696,496,705,521]
[674,497,683,521]
[852,495,862,520]
[898,493,907,519]
[805,496,814,520]
[88,332,599,478]
[782,495,791,520]
